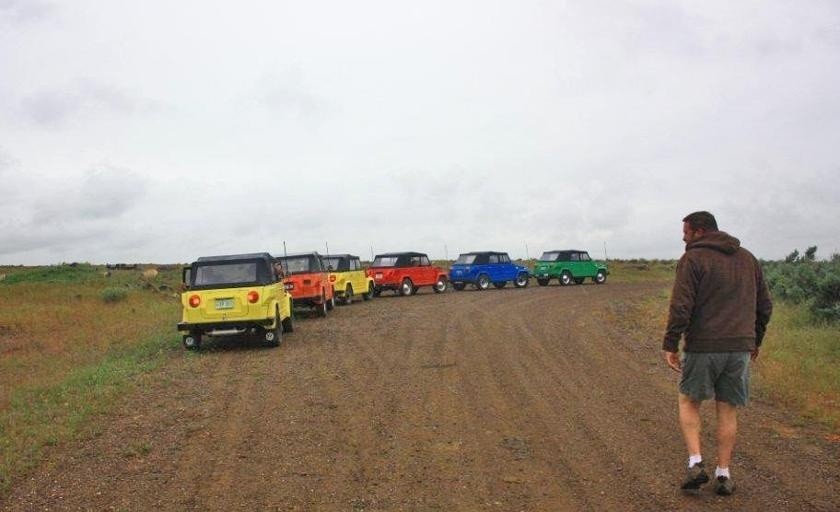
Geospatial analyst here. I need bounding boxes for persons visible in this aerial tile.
[663,211,773,495]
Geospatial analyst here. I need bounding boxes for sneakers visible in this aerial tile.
[681,460,709,490]
[714,476,735,496]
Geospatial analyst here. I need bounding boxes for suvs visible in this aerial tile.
[531,250,608,286]
[275,253,333,317]
[367,253,448,297]
[319,253,377,306]
[448,252,530,291]
[177,254,294,345]
